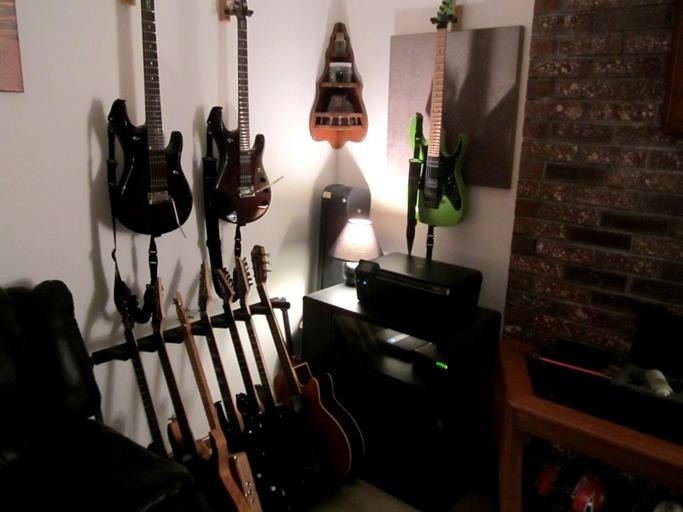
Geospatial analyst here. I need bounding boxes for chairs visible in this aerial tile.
[1,280,195,512]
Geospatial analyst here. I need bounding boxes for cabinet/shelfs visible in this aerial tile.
[299,282,501,512]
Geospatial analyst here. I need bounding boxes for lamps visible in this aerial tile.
[316,184,385,291]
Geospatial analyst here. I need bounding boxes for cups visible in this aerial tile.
[641,369,674,398]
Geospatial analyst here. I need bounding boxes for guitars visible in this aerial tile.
[114,281,215,511]
[146,279,256,511]
[213,268,320,511]
[109,1,192,235]
[231,256,333,510]
[199,262,299,511]
[405,0,467,227]
[171,297,264,510]
[249,245,365,490]
[209,0,270,223]
